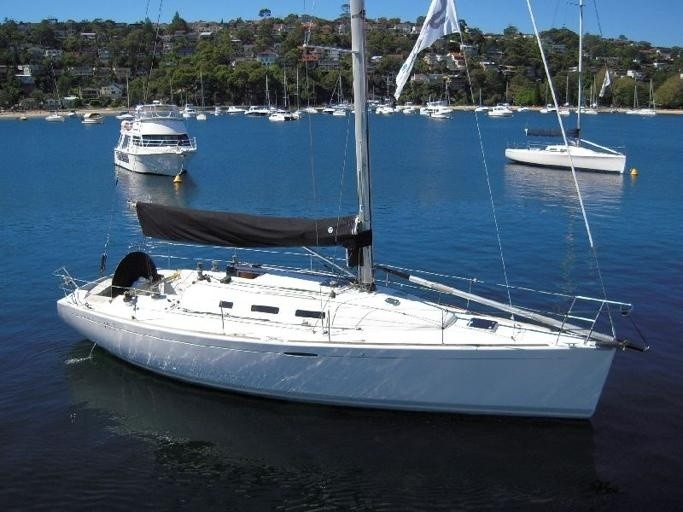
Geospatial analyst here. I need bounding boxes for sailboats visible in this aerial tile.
[56,0,650,418]
[504,0,627,173]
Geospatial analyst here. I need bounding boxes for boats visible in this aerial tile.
[183,101,355,121]
[45,115,65,121]
[369,97,454,118]
[81,111,102,124]
[475,106,514,118]
[113,99,198,176]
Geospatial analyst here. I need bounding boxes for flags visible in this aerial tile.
[599,70,611,97]
[391,0,461,104]
